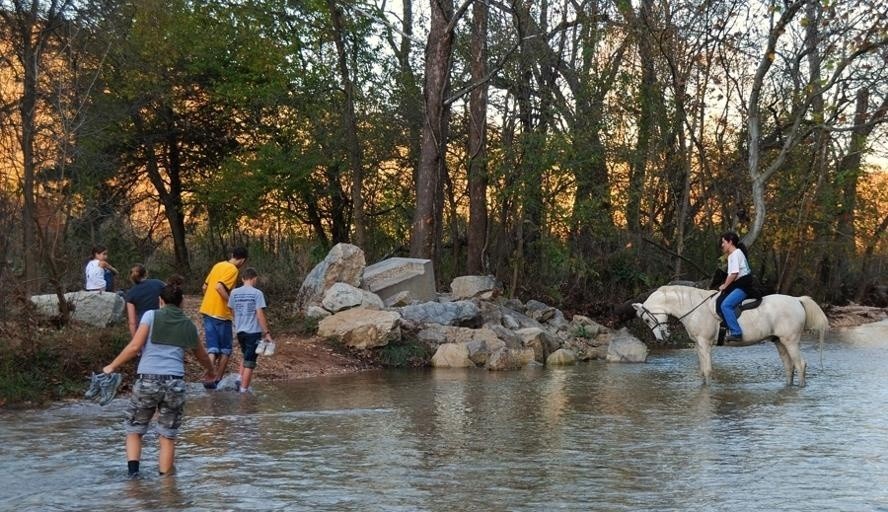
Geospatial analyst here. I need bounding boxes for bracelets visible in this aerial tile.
[265,330,269,336]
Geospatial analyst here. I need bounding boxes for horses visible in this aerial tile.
[631,284,830,389]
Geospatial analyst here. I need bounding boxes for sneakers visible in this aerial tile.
[263,341,275,356]
[84,372,111,400]
[255,338,267,354]
[98,372,122,406]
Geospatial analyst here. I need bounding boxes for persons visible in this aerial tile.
[718,232,752,342]
[103,282,219,478]
[125,262,167,357]
[200,246,249,389]
[226,265,274,393]
[85,244,109,292]
[85,256,120,292]
